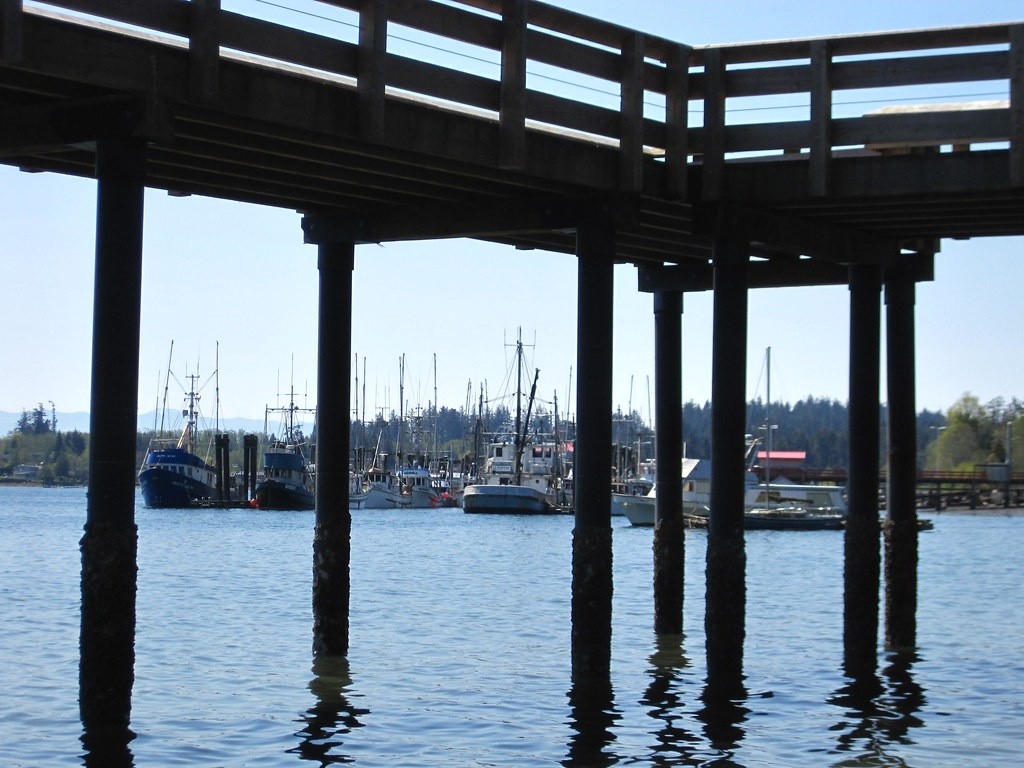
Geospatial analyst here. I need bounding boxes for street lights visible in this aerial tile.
[932,426,948,472]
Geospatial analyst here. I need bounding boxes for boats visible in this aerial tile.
[138,338,846,529]
